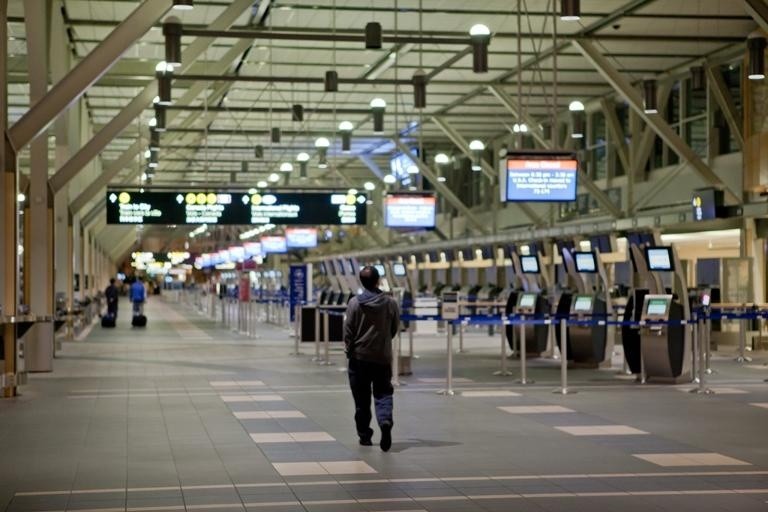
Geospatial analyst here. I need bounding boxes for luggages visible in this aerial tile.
[132,315,146,327]
[102,314,116,327]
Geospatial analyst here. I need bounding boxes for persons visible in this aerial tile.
[101,274,161,327]
[341,264,403,453]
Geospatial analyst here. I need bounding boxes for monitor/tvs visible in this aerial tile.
[645,245,675,273]
[520,255,542,275]
[645,297,669,319]
[692,187,725,220]
[393,263,407,277]
[572,250,598,274]
[520,293,534,311]
[373,264,387,278]
[219,270,284,279]
[574,295,592,312]
[315,257,357,276]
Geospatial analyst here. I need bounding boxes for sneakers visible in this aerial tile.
[359,427,373,446]
[379,423,392,452]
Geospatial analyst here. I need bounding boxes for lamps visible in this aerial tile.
[639,73,660,117]
[470,32,488,72]
[568,105,586,141]
[744,35,768,81]
[558,1,582,21]
[143,15,182,193]
[227,0,432,187]
